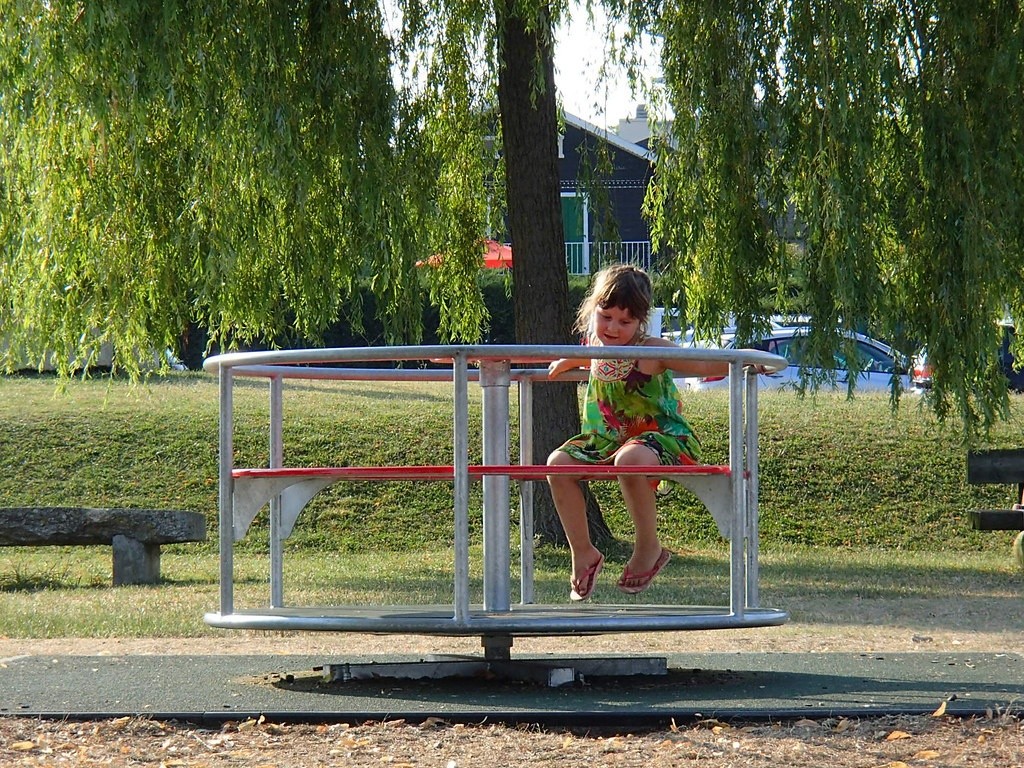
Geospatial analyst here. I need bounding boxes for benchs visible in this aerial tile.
[966,448,1023,531]
[0,507,206,585]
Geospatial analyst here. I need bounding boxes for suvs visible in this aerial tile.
[909,319,1024,396]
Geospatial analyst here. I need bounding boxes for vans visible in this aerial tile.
[644,307,787,345]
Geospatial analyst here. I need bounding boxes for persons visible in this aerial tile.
[544,264,768,602]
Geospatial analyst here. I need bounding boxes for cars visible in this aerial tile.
[678,325,915,390]
[762,313,851,330]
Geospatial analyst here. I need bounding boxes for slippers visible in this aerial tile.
[616,547,671,594]
[570,553,605,601]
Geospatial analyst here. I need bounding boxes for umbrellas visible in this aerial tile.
[414,238,514,269]
